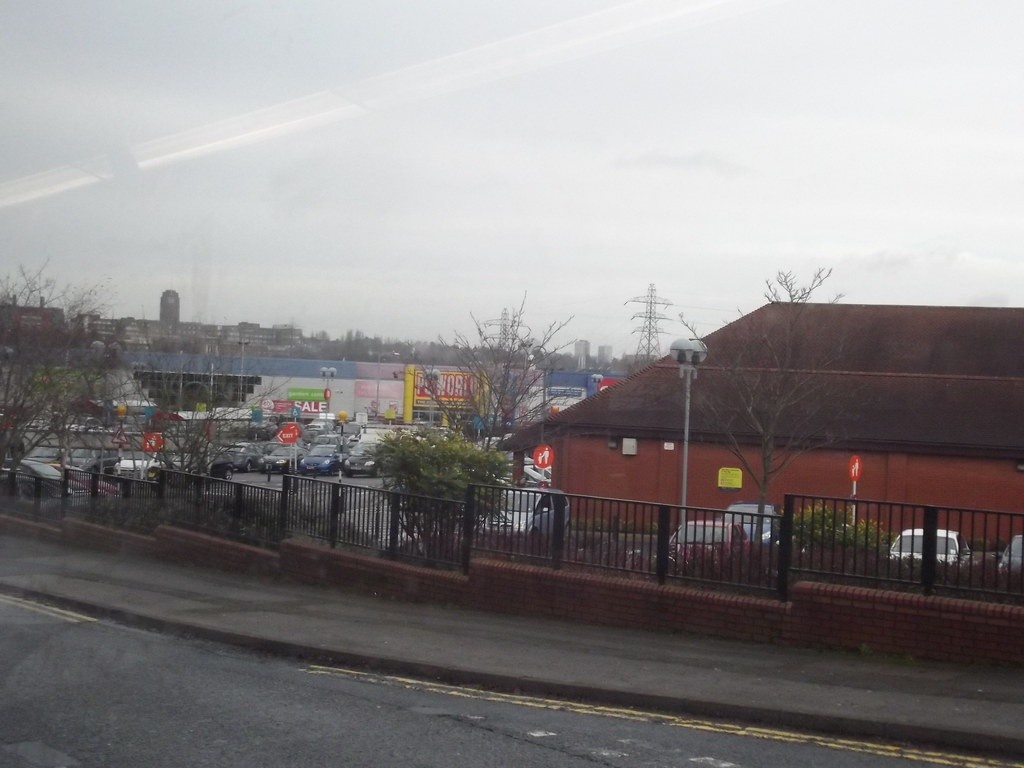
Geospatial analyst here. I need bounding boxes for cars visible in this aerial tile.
[887,527,974,571]
[479,488,571,539]
[722,502,777,547]
[991,534,1023,580]
[668,519,750,581]
[1,416,553,512]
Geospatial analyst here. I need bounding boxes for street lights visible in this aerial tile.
[527,345,547,482]
[376,351,402,416]
[590,373,603,394]
[318,366,337,437]
[670,337,710,552]
[422,369,440,428]
